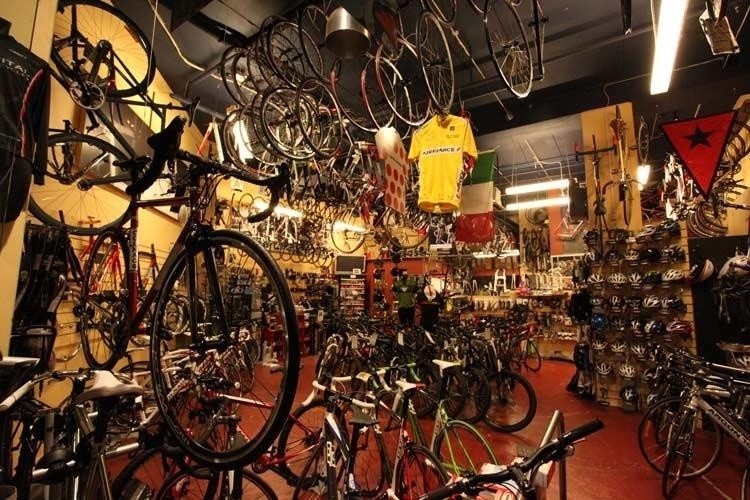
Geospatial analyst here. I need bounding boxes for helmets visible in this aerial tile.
[589,294,685,312]
[587,268,685,289]
[583,219,681,248]
[647,394,658,406]
[589,314,693,336]
[591,338,656,361]
[582,244,687,268]
[595,362,653,383]
[620,384,639,402]
[718,256,749,283]
[691,258,715,283]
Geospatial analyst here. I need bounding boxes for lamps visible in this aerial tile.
[505,138,572,213]
[649,0,690,97]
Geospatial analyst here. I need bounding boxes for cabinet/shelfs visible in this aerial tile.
[339,275,368,320]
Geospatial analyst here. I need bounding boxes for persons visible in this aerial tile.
[391,272,418,325]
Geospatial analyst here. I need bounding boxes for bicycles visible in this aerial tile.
[0,158,606,499]
[637,337,750,497]
[575,104,749,236]
[216,0,550,182]
[23,1,201,237]
[74,114,302,472]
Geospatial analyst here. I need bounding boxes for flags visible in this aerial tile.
[455,147,496,246]
